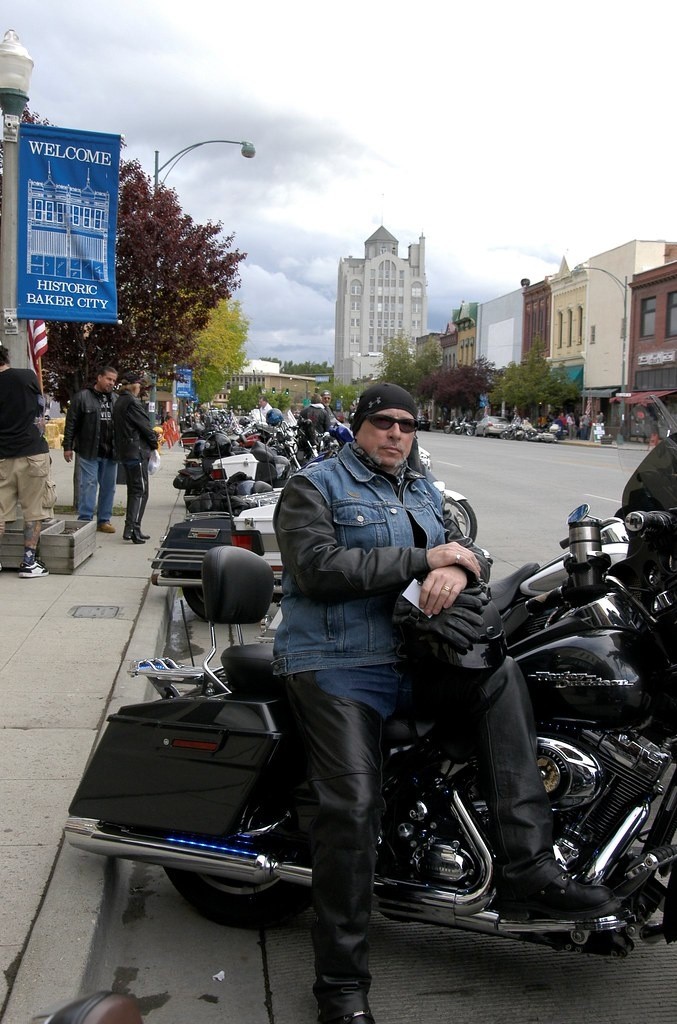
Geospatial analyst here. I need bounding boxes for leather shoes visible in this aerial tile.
[317,1004,376,1024]
[515,872,620,916]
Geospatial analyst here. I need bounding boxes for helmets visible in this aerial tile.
[204,433,230,457]
[266,408,282,425]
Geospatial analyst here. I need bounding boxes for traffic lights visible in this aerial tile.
[285,389,289,395]
[272,386,275,394]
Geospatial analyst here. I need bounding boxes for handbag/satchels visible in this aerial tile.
[148,449,159,476]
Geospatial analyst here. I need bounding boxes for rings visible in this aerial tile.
[455,554,460,564]
[442,585,451,592]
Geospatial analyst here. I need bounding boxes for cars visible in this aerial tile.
[474,416,510,437]
[417,416,430,431]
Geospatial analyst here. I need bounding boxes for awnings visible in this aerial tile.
[581,388,619,398]
[609,389,677,407]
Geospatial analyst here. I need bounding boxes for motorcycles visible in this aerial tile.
[179,408,478,621]
[64,396,677,959]
[444,416,477,436]
[500,414,538,441]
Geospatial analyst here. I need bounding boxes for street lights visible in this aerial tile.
[0,30,36,370]
[290,377,308,399]
[341,358,361,379]
[149,140,255,431]
[571,266,627,446]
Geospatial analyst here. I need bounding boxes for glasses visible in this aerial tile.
[366,414,419,432]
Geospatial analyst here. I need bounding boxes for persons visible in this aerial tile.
[546,410,604,440]
[165,412,173,421]
[298,389,336,433]
[258,396,272,418]
[0,344,57,577]
[271,381,622,1024]
[111,371,163,543]
[62,367,119,533]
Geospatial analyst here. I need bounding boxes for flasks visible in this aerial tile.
[568,520,601,588]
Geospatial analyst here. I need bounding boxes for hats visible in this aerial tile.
[350,382,419,435]
[122,372,144,382]
[320,390,331,397]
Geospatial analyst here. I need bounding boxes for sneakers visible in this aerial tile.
[18,559,49,577]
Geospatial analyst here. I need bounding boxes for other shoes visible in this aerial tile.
[97,522,115,533]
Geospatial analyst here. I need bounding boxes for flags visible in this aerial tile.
[26,319,48,378]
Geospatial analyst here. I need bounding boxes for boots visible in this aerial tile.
[139,523,150,539]
[123,521,145,544]
